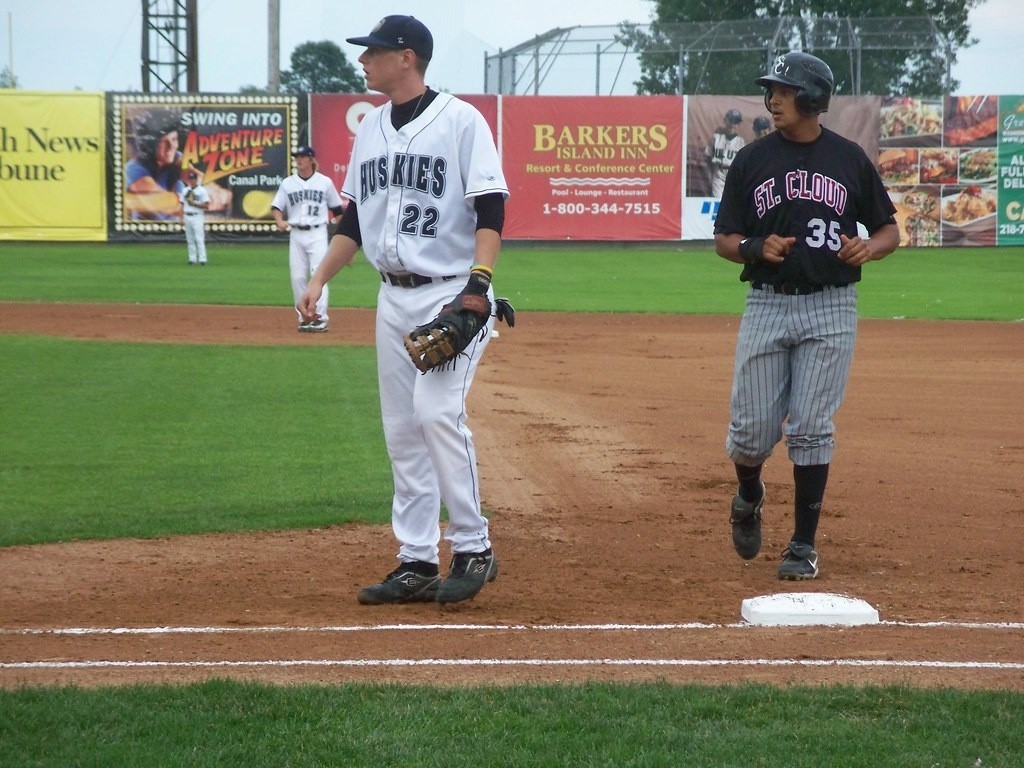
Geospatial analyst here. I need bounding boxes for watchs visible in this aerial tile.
[737,238,748,251]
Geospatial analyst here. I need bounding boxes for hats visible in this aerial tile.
[187,172,197,179]
[289,145,315,157]
[346,14,432,60]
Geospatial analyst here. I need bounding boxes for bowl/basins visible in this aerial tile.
[941,192,996,228]
[880,104,941,148]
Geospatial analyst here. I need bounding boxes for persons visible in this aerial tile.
[753,116,772,139]
[270,146,354,331]
[176,172,211,267]
[713,49,901,581]
[297,15,511,608]
[706,108,745,198]
[126,112,233,220]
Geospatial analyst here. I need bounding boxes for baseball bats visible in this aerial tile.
[121,189,233,213]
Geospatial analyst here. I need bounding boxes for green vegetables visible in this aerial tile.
[959,147,1000,179]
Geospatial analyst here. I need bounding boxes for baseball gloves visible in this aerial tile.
[184,191,196,202]
[402,287,497,375]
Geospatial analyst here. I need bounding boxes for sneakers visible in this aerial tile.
[776,540,820,581]
[309,321,328,333]
[729,480,766,563]
[434,545,497,611]
[297,321,312,333]
[356,561,441,605]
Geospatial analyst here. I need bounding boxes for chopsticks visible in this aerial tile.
[966,95,987,113]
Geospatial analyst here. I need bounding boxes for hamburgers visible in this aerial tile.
[876,150,909,183]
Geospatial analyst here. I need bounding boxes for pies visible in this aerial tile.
[889,189,941,247]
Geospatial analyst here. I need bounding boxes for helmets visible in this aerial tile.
[754,51,835,116]
[724,108,743,121]
[135,110,188,153]
[752,116,771,128]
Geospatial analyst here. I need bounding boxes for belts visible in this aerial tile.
[289,224,319,230]
[184,212,199,215]
[380,270,456,290]
[749,278,856,296]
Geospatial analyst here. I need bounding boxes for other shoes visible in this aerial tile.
[199,262,207,265]
[187,261,195,266]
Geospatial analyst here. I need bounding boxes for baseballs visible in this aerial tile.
[242,190,270,219]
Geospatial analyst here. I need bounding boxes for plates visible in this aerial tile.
[959,147,997,183]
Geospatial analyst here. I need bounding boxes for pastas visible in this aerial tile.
[881,103,942,138]
